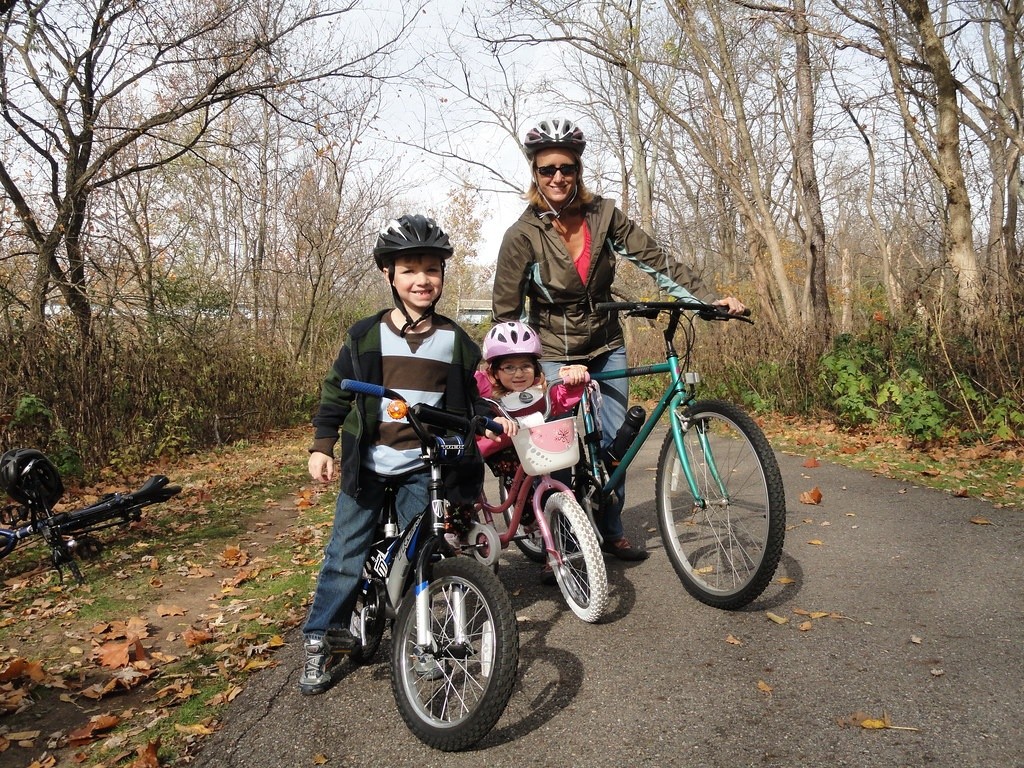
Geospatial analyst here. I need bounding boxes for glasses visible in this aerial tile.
[536,165,577,176]
[498,364,533,375]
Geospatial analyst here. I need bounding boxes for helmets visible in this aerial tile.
[482,322,543,361]
[373,214,453,273]
[524,120,586,156]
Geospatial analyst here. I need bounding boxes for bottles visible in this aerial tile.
[613,406,647,459]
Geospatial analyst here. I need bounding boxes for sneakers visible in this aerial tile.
[299,632,344,695]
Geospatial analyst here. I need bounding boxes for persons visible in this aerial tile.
[444,321,586,582]
[493,116,748,580]
[299,213,520,696]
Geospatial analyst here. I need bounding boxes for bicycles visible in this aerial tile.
[322,378,522,753]
[1,457,183,587]
[473,372,610,624]
[496,297,789,612]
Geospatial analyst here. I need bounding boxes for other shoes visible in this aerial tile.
[600,537,648,561]
[541,557,556,583]
